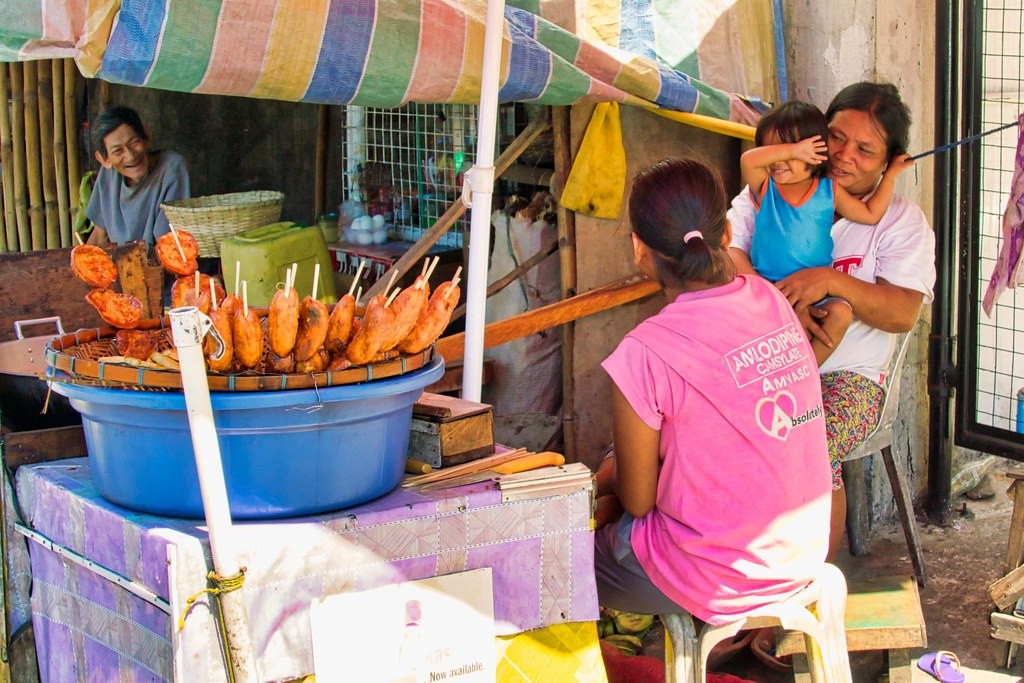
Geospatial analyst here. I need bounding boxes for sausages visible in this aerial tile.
[185,276,460,374]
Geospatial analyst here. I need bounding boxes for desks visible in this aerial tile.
[14,444,609,683]
[327,239,466,338]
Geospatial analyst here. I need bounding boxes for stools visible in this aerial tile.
[659,563,853,683]
[775,573,928,683]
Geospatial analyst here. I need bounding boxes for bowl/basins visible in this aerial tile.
[47,356,444,518]
[343,225,390,246]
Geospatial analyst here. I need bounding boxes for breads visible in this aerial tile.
[98,349,181,371]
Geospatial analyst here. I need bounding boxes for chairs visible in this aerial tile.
[841,329,928,589]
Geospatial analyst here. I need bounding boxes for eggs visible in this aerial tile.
[347,214,387,245]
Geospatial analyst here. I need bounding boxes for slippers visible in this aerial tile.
[917,650,965,683]
[750,623,793,672]
[707,627,754,670]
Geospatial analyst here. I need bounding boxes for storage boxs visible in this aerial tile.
[408,390,496,468]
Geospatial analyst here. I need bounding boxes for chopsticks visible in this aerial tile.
[401,447,528,488]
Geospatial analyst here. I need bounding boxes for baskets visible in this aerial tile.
[160,190,285,258]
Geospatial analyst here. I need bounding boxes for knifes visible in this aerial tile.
[422,452,564,490]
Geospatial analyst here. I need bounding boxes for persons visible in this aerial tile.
[592,158,832,626]
[84,106,211,306]
[706,83,935,672]
[741,101,915,369]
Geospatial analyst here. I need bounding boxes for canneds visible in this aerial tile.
[317,212,338,243]
